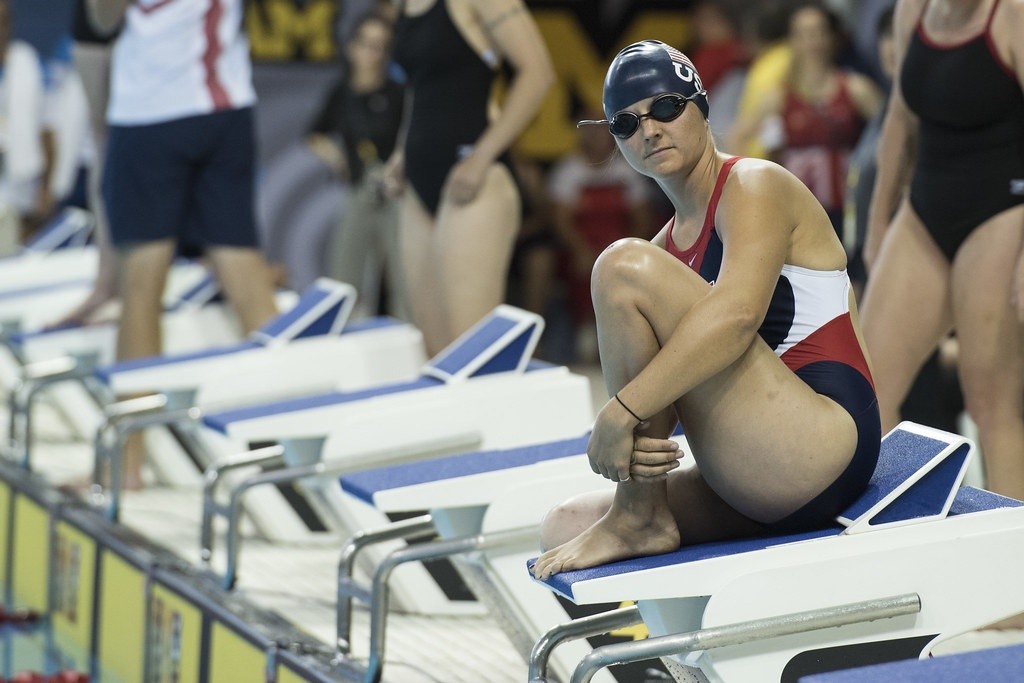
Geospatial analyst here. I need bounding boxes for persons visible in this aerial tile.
[0,0,275,488]
[861,0,1023,633]
[531,40,882,581]
[306,0,903,362]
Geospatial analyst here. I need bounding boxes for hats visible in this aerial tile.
[602,39,709,117]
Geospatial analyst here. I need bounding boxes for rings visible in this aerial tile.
[620,477,631,482]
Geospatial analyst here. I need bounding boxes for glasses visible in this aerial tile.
[609,89,705,139]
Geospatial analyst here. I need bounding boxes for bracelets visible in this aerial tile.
[615,393,643,422]
